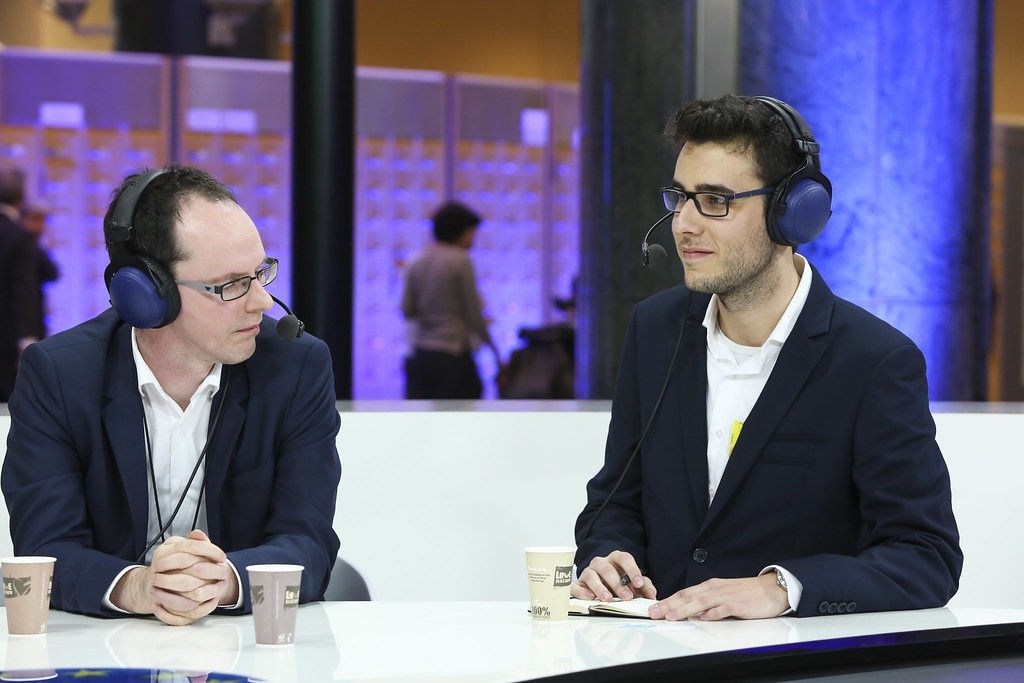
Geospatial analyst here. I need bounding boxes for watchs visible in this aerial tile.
[774,568,788,591]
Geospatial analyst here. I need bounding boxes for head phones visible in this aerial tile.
[104,168,182,330]
[750,96,833,246]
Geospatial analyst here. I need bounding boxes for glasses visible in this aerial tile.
[659,185,776,217]
[175,256,279,301]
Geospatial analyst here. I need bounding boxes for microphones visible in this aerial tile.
[267,293,304,339]
[641,212,673,272]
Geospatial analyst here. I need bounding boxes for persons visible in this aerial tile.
[0,168,57,404]
[399,197,494,399]
[573,93,964,619]
[0,162,343,625]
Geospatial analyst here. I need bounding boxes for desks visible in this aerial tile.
[0,598,1024,683]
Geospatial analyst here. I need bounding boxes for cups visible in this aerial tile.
[525,546,578,620]
[248,644,297,683]
[245,564,305,648]
[0,634,58,682]
[1,556,57,634]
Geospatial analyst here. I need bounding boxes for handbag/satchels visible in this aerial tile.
[494,322,574,400]
